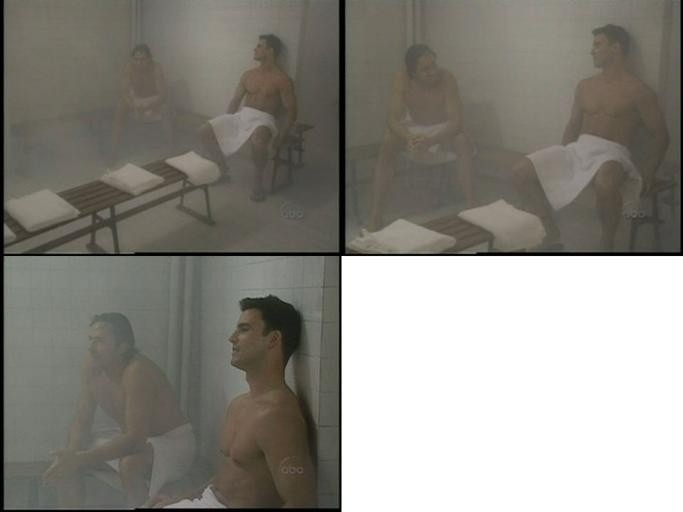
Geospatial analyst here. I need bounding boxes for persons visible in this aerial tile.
[360,43,475,233]
[180,294,318,509]
[201,33,300,206]
[40,310,194,509]
[104,44,178,165]
[512,24,670,253]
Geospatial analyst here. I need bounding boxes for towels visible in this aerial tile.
[398,112,477,165]
[127,89,162,122]
[524,132,641,217]
[105,419,227,511]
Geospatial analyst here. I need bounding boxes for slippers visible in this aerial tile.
[249,187,264,202]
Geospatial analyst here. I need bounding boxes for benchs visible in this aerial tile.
[5,462,209,510]
[345,138,676,254]
[3,106,313,254]
